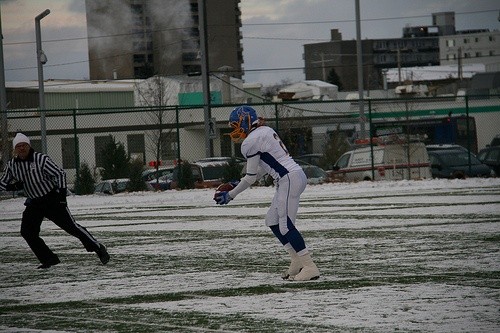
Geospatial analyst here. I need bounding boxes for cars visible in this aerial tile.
[93,179,154,194]
[426,144,495,179]
[148,173,177,190]
[142,165,175,180]
[300,165,329,184]
[476,146,496,177]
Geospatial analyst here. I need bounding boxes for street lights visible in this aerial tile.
[34,10,49,154]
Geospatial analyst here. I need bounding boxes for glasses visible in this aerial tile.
[16,143,29,148]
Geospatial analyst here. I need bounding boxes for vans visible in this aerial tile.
[323,144,426,182]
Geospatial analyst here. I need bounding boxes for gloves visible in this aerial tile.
[56,188,67,208]
[6,180,25,191]
[214,191,233,205]
[230,178,241,187]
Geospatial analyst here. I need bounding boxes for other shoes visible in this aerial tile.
[36,254,61,269]
[94,243,110,264]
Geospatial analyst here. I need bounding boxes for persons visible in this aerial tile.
[213,105,320,281]
[0,133,110,269]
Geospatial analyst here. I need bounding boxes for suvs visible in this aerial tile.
[169,161,235,190]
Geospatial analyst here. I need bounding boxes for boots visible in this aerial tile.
[288,247,320,280]
[281,242,304,280]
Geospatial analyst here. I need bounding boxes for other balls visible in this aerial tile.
[215,183,234,205]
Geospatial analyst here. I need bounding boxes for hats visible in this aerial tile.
[13,133,31,152]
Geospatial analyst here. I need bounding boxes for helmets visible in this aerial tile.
[229,106,259,144]
